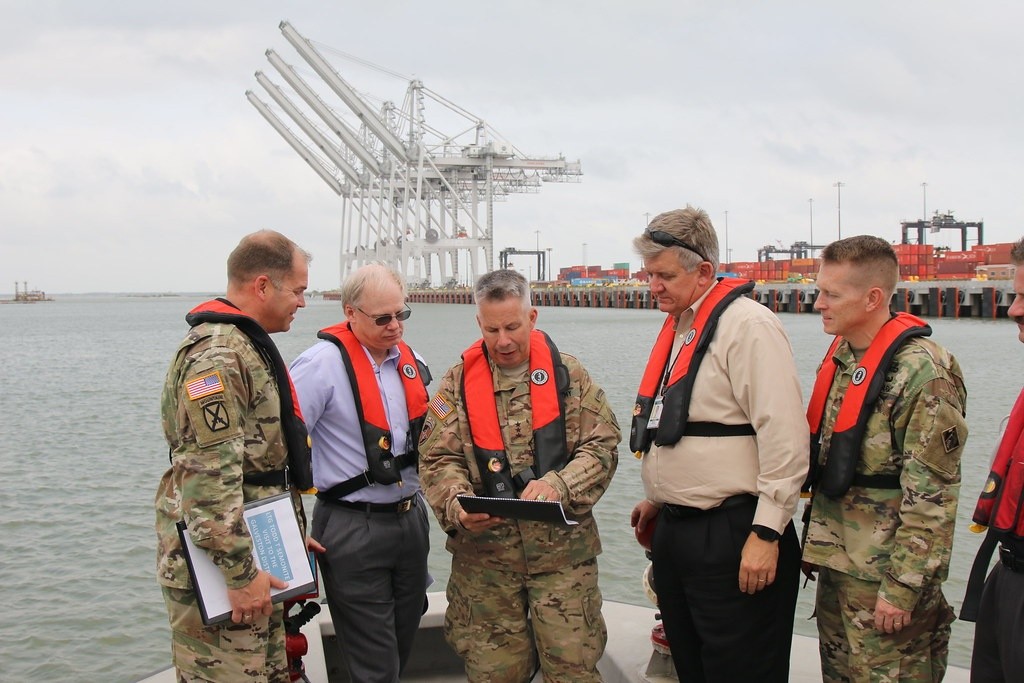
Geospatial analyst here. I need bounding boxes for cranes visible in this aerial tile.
[242,17,586,289]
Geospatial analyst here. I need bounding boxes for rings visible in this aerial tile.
[759,578,766,582]
[243,614,253,618]
[537,495,546,502]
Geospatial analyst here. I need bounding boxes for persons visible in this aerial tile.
[804,234,968,683]
[286,266,437,683]
[419,269,623,682]
[154,229,327,682]
[959,236,1024,683]
[630,206,812,683]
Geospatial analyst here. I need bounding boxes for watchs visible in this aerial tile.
[751,525,781,542]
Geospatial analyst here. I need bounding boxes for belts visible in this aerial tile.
[243,468,293,486]
[336,493,418,514]
[998,546,1024,572]
[666,496,750,517]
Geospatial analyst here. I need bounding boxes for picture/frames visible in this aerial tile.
[173,491,316,625]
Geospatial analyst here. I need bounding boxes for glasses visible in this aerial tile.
[357,303,412,326]
[645,227,707,261]
